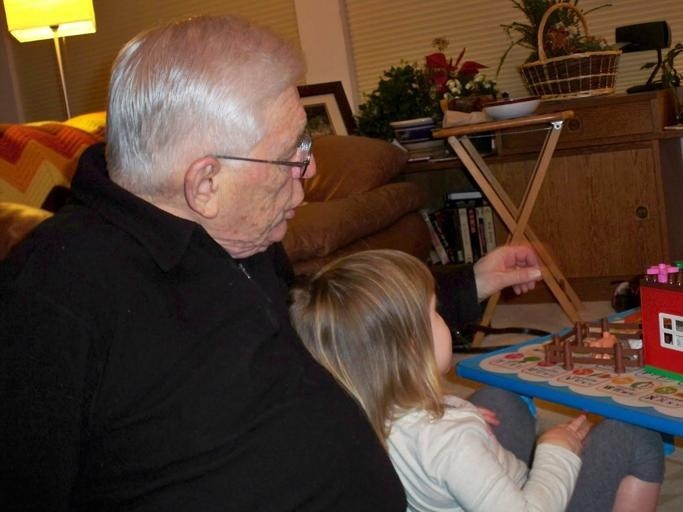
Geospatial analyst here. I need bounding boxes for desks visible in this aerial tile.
[432,109,583,343]
[453,307,682,455]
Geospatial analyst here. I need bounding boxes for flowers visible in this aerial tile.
[417,35,495,94]
[504,2,612,59]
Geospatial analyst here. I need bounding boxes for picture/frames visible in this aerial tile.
[292,78,360,140]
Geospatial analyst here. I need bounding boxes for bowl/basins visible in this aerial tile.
[482,95,542,122]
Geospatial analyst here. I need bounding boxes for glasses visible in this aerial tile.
[214,127,312,177]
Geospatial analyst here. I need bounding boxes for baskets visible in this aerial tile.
[515,1,622,102]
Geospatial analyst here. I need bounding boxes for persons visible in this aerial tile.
[291,248,665,511]
[0,18,544,510]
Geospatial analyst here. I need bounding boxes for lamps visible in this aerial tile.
[0,0,113,132]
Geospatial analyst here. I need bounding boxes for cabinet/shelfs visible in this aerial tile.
[478,94,683,303]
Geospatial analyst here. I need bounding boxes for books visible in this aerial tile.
[386,115,452,163]
[416,191,497,266]
